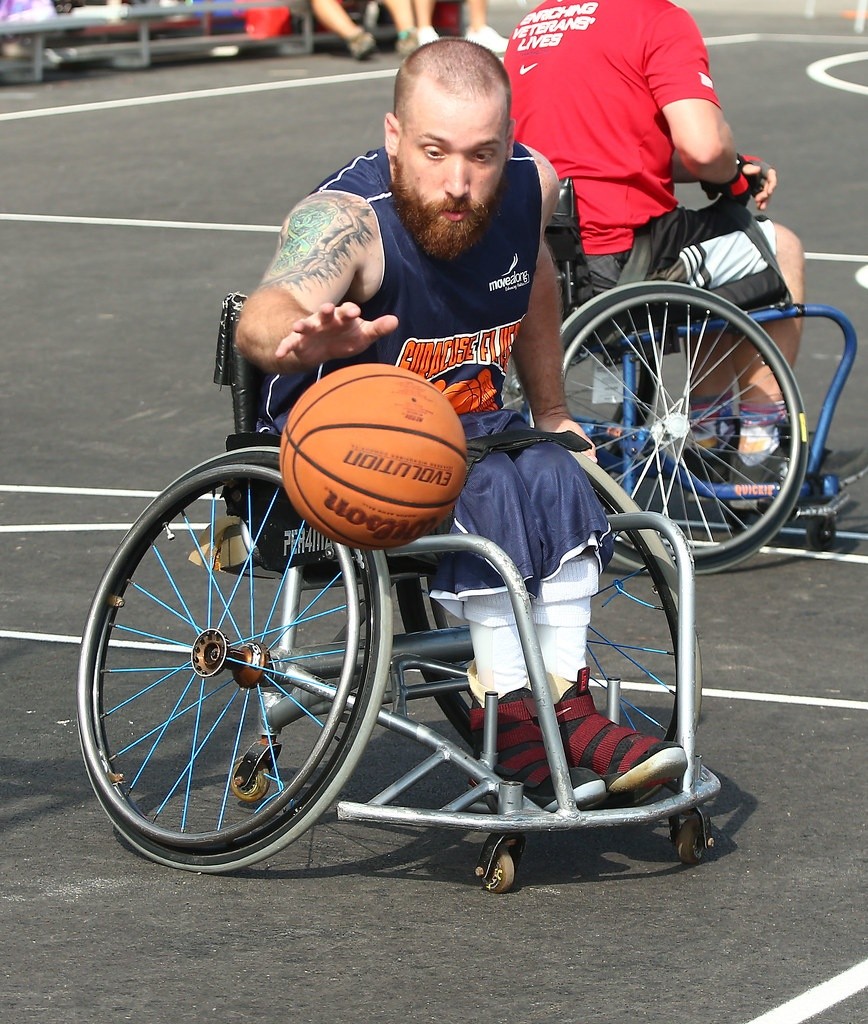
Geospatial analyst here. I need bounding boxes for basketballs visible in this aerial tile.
[280,364,465,547]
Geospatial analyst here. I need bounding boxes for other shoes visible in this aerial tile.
[396,26,422,57]
[346,27,376,60]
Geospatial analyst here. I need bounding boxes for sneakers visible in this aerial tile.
[418,26,439,47]
[464,24,510,53]
[680,432,868,510]
[553,666,689,792]
[466,687,608,813]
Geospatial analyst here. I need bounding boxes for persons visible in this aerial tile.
[235,36,688,811]
[310,1,510,54]
[503,0,868,488]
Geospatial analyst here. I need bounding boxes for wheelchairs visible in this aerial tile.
[529,174,861,575]
[72,289,724,892]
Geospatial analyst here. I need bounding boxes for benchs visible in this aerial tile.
[1,0,467,83]
